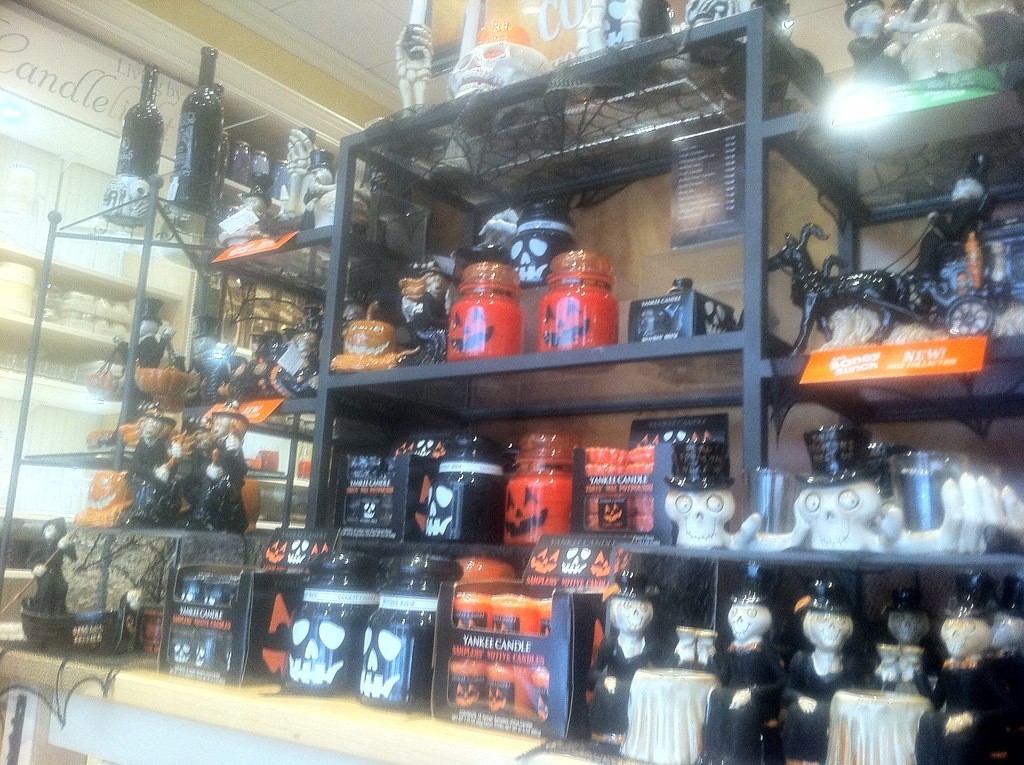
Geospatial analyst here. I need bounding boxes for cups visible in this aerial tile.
[747,468,803,535]
[890,450,968,529]
[802,427,873,470]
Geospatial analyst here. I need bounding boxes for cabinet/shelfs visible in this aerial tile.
[0,0,1024,765]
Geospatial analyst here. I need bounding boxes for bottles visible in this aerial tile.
[101,64,164,225]
[271,128,317,199]
[169,46,223,212]
[301,151,334,227]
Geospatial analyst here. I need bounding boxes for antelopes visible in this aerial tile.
[768,223,910,356]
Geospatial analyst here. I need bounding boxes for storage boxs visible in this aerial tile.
[333,454,441,544]
[627,291,733,344]
[430,579,606,745]
[156,564,307,689]
